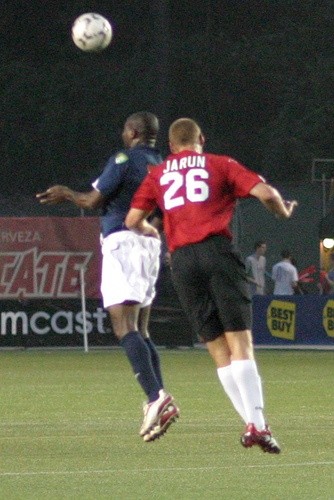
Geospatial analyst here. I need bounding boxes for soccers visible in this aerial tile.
[71,12,112,52]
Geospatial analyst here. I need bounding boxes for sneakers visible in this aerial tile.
[240,423,281,454]
[138,389,181,442]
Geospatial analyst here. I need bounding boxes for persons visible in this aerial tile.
[161,251,173,306]
[36,112,181,443]
[272,250,299,295]
[243,240,272,295]
[125,118,299,454]
